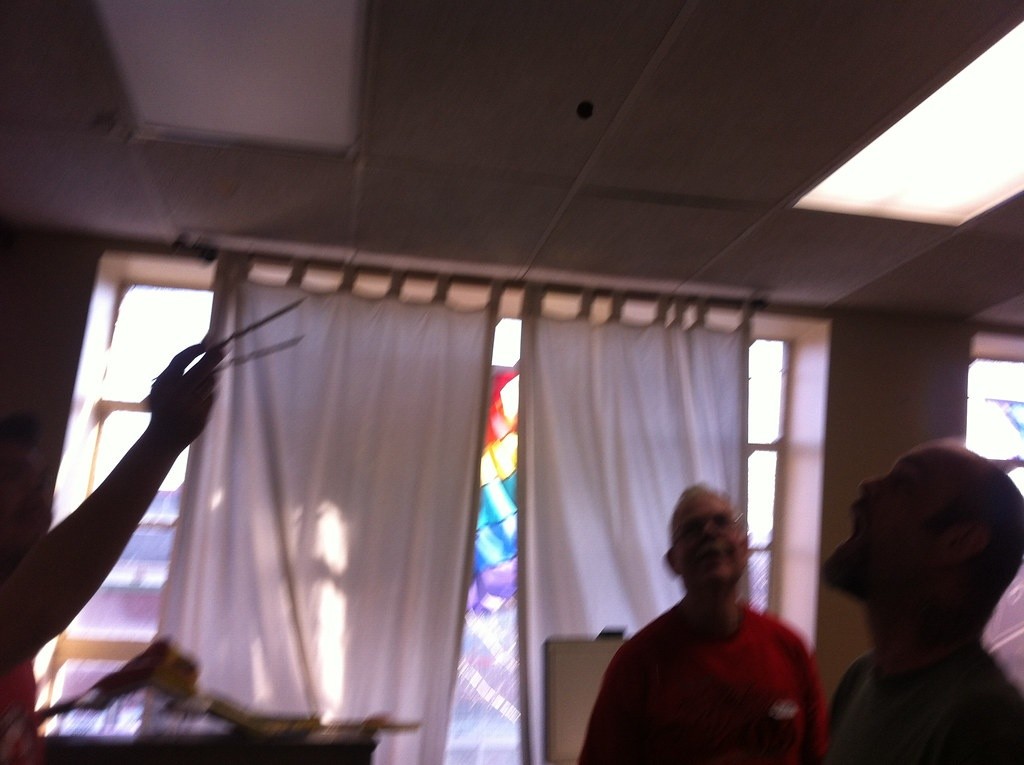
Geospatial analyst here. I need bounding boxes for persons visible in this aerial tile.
[823,440,1024,765]
[0,342,229,765]
[576,483,828,765]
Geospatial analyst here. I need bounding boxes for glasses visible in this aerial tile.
[672,516,733,548]
